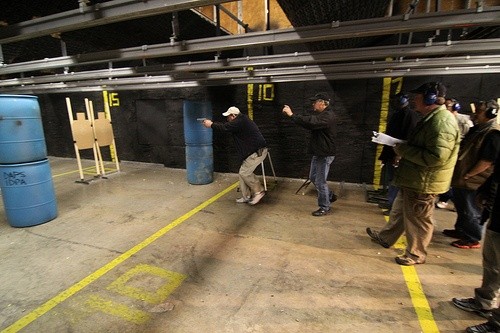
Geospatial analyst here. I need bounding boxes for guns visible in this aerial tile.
[196,117,209,122]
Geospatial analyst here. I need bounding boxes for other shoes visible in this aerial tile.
[435,200,447,209]
[236,196,249,203]
[312,206,331,216]
[329,193,338,203]
[248,192,265,206]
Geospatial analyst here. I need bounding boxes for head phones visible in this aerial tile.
[451,98,461,110]
[486,104,499,119]
[400,92,409,105]
[424,82,439,106]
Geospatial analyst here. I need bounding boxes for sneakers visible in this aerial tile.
[442,228,464,239]
[451,238,481,249]
[452,296,493,317]
[367,226,389,248]
[395,255,426,266]
[467,314,500,333]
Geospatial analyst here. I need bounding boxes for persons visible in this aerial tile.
[203,106,268,205]
[282,92,338,216]
[366,80,460,265]
[378,88,500,249]
[453,153,500,333]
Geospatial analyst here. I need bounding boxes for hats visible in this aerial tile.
[410,81,446,97]
[222,106,240,116]
[309,92,330,102]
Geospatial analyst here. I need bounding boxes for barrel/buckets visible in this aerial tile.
[0,158,58,228]
[0,94,47,164]
[183,100,213,143]
[185,143,214,184]
